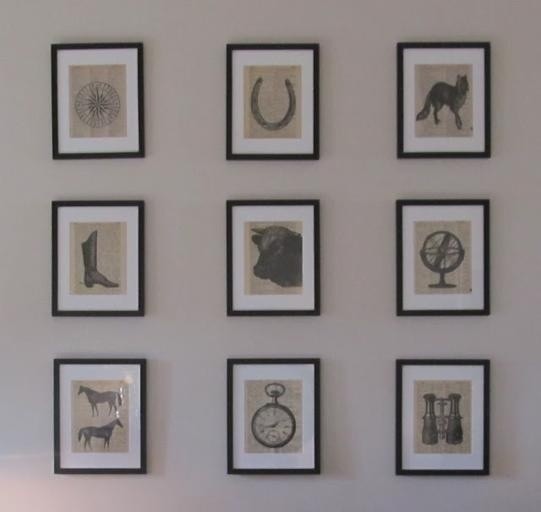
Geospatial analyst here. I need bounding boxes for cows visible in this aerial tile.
[249,223,302,290]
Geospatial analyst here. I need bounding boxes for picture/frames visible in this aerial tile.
[398,42,488,158]
[228,43,321,161]
[397,357,490,475]
[396,200,492,316]
[51,199,145,317]
[229,358,322,475]
[52,357,150,474]
[226,199,320,317]
[51,42,146,160]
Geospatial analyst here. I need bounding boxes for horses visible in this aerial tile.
[77,417,124,450]
[77,385,124,416]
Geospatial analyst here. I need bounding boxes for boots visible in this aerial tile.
[82,231,119,288]
